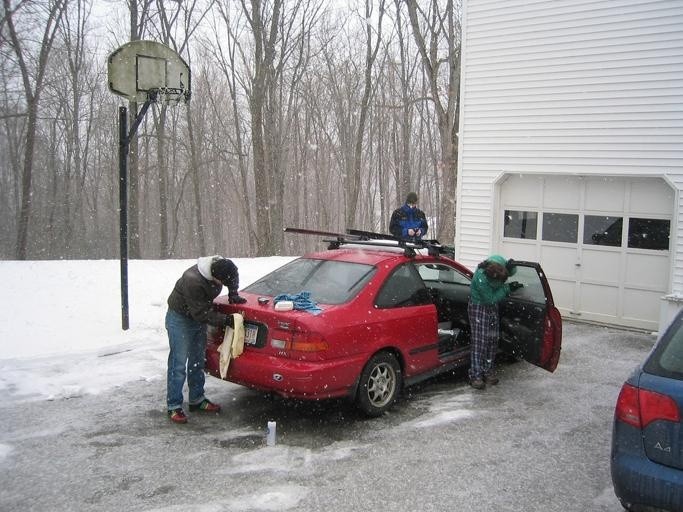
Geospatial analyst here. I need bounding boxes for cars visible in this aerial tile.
[609,307,682,512]
[204,228,562,418]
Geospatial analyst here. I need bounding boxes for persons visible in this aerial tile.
[465,256,525,389]
[389,192,428,241]
[163,252,247,423]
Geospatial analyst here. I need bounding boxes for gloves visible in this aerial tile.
[509,281,523,292]
[225,314,234,330]
[506,259,517,270]
[229,293,248,304]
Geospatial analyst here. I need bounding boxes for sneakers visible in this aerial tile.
[189,399,220,412]
[469,378,485,389]
[168,408,187,424]
[484,375,499,385]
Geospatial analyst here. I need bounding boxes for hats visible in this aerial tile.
[407,192,417,202]
[212,259,238,286]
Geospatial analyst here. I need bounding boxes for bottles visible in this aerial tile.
[267,418,276,447]
